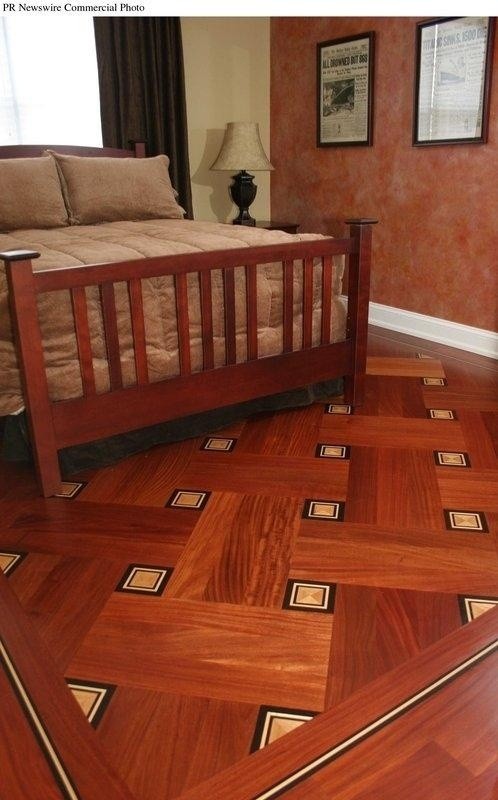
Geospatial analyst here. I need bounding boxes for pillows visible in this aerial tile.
[48,149,184,226]
[0,151,69,231]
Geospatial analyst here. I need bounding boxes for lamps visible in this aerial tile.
[209,122,276,226]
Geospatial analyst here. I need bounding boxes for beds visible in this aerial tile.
[0,143,380,498]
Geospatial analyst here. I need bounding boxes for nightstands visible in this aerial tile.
[225,220,301,234]
